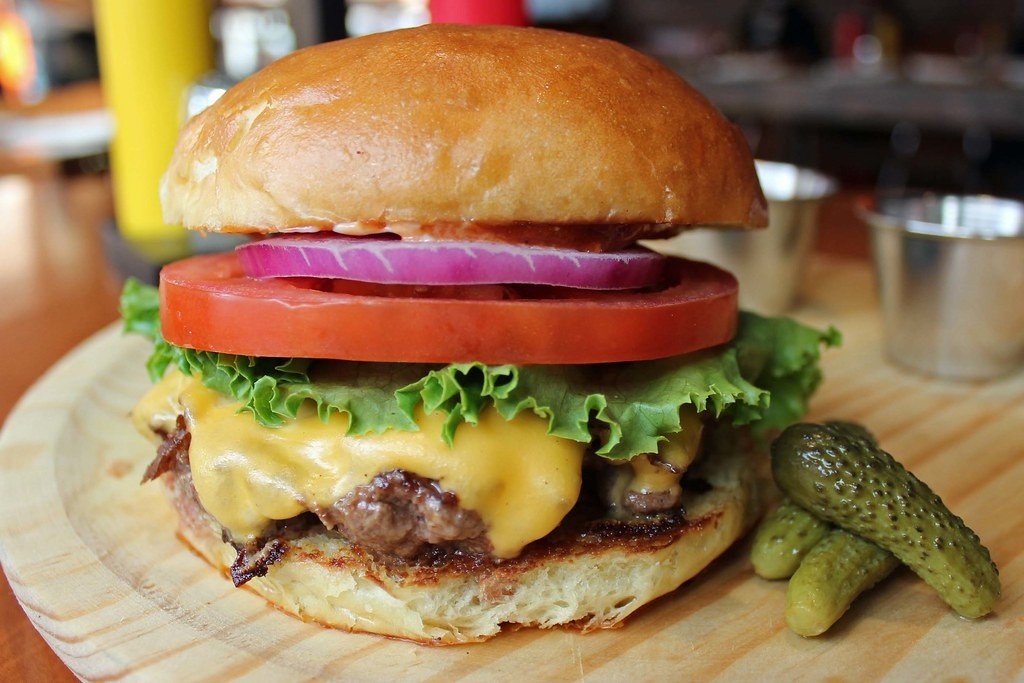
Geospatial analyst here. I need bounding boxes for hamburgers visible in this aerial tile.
[121,21,841,651]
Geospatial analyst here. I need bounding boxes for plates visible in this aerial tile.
[0,250,1023,678]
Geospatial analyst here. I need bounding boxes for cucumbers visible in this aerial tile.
[751,423,998,637]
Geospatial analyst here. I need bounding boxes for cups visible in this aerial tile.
[630,157,842,320]
[852,193,1024,381]
